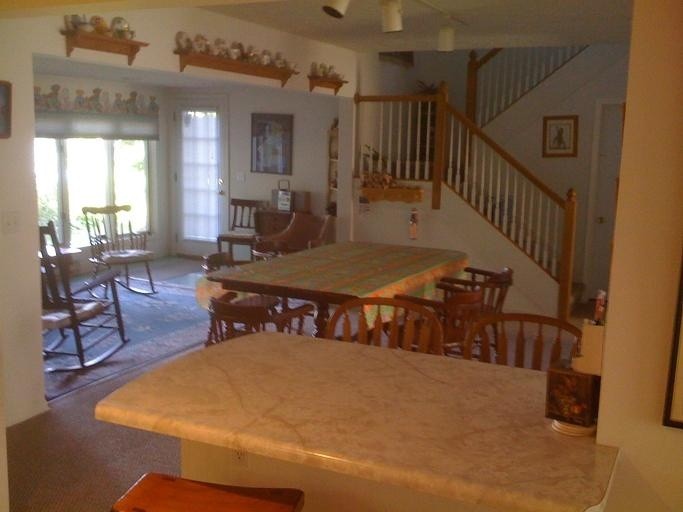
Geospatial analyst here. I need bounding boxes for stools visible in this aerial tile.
[110,469,308,512]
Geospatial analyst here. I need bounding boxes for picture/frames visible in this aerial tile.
[250,111,293,175]
[1,76,15,142]
[660,260,683,430]
[540,114,582,160]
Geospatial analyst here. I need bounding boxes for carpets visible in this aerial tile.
[43,271,246,400]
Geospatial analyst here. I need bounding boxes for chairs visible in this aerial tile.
[385,281,486,358]
[440,264,517,349]
[322,296,446,358]
[80,203,158,300]
[204,288,315,345]
[216,198,266,264]
[252,211,331,261]
[461,312,587,371]
[203,250,237,272]
[39,219,130,377]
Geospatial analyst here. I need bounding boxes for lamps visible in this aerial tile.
[321,0,456,53]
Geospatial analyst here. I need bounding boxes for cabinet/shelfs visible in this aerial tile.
[324,123,339,218]
[253,208,314,233]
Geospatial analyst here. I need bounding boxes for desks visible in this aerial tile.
[202,241,472,338]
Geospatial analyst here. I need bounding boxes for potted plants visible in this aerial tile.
[415,80,436,106]
